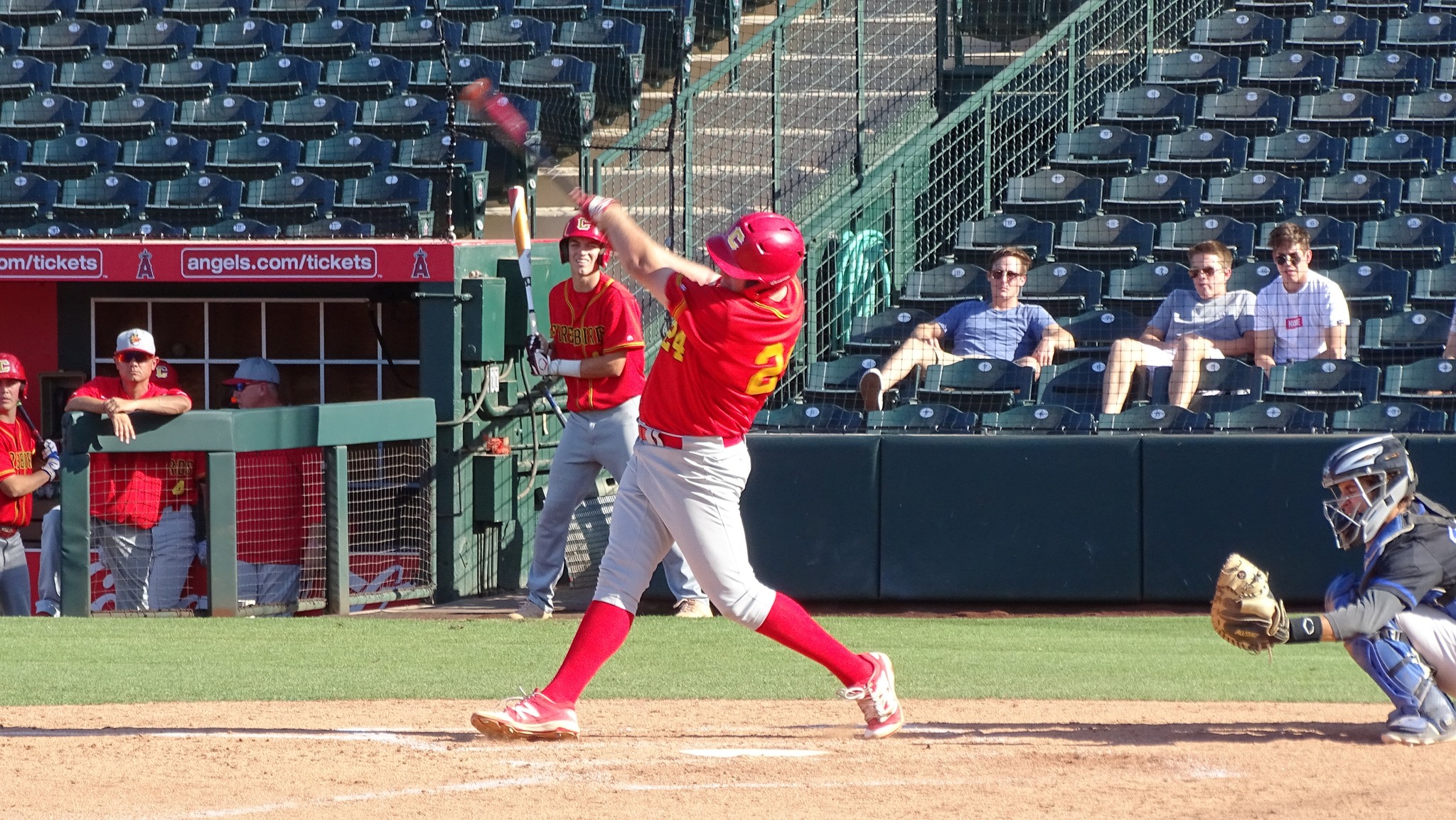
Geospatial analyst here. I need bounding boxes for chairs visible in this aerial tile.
[0,0,1456,434]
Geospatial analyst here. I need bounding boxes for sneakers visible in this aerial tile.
[836,652,904,738]
[469,687,580,740]
[1379,713,1455,745]
[674,597,714,618]
[507,599,553,620]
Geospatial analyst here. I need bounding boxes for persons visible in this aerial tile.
[1253,224,1350,395]
[224,357,323,617]
[860,247,1074,412]
[1101,241,1256,414]
[1211,435,1456,744]
[1417,308,1456,395]
[508,213,713,621]
[150,362,206,609]
[0,353,59,617]
[34,328,191,616]
[470,196,904,740]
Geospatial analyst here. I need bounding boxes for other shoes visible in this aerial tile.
[861,368,883,412]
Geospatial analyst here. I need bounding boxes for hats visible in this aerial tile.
[113,329,155,356]
[223,356,280,385]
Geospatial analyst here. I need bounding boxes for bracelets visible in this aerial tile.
[1285,616,1323,643]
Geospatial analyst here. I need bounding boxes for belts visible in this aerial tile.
[638,425,743,448]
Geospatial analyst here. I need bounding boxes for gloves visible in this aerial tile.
[526,351,552,377]
[525,332,551,357]
[41,438,58,459]
[581,194,621,222]
[41,455,60,481]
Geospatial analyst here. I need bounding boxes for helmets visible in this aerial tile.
[559,214,611,268]
[149,360,182,390]
[0,353,27,399]
[706,213,805,301]
[1321,434,1416,550]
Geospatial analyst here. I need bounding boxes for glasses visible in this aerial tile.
[116,354,151,362]
[233,384,250,391]
[1275,253,1306,265]
[991,269,1024,280]
[1188,267,1223,278]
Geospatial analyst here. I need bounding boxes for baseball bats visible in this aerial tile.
[17,399,46,450]
[460,79,590,208]
[507,186,538,336]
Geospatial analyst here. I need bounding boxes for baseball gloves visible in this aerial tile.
[1210,553,1289,651]
